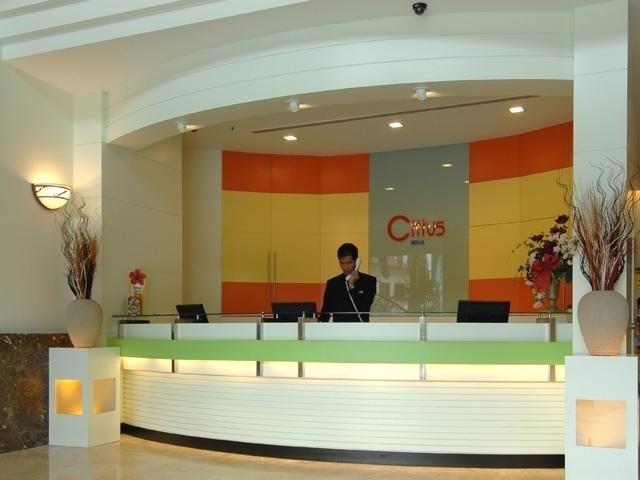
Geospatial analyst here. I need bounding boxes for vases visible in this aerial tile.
[547,278,560,311]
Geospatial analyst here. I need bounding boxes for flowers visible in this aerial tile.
[506,214,579,309]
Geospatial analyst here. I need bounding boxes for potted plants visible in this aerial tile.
[55,189,106,348]
[552,153,640,356]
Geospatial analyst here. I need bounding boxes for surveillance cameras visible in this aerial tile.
[412,2,427,17]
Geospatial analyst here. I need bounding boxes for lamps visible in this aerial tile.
[31,182,72,210]
[176,85,430,135]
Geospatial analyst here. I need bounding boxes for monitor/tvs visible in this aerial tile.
[175,303,208,323]
[456,300,512,323]
[270,302,317,321]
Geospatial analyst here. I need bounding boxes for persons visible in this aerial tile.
[316,241,379,321]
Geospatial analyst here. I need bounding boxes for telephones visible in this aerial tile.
[345,259,361,281]
